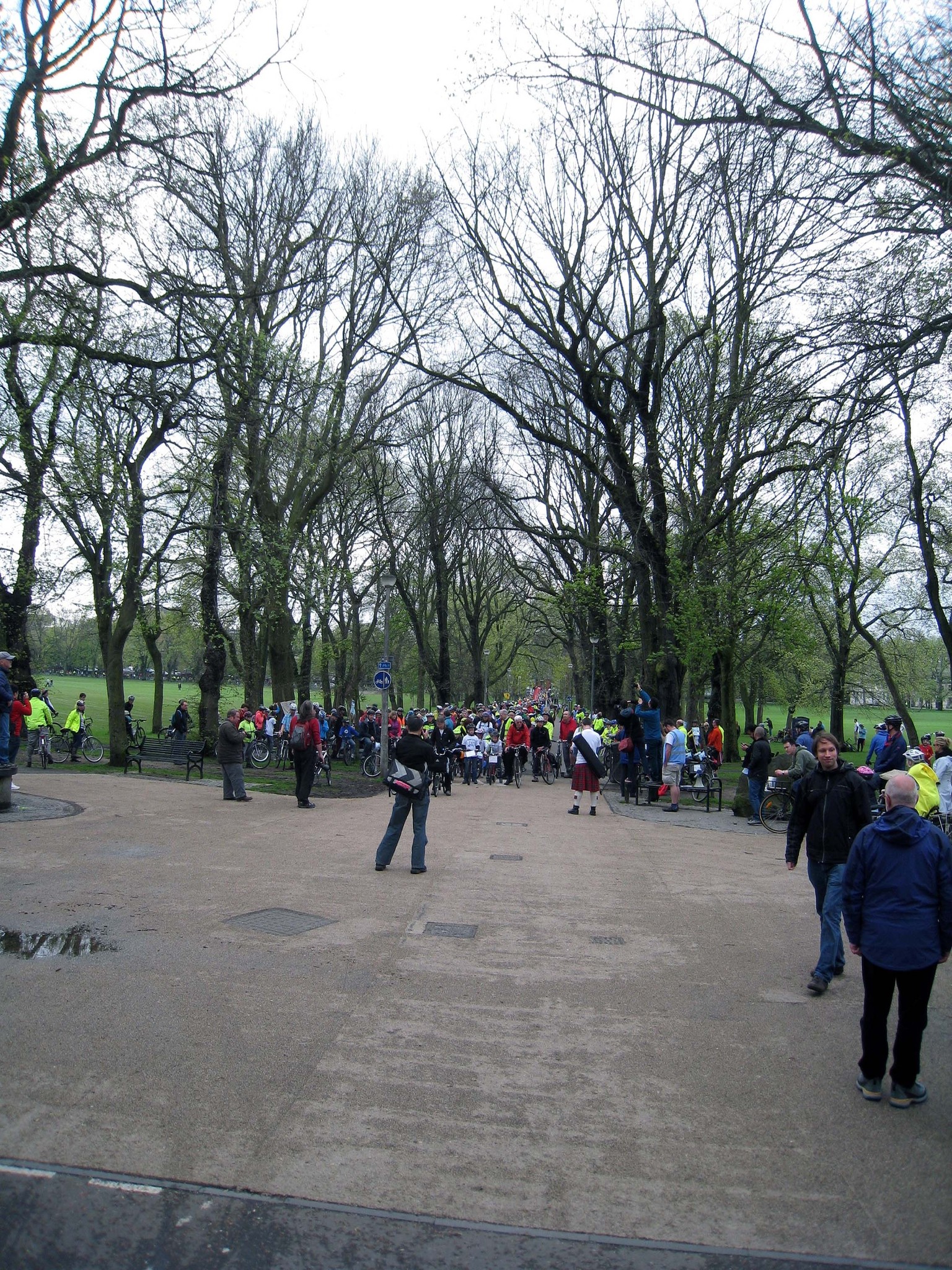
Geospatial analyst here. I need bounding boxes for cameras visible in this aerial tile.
[631,683,638,688]
[614,704,621,709]
[420,729,426,735]
[315,757,330,772]
[238,728,252,738]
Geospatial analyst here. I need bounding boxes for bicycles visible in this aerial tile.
[1,634,952,861]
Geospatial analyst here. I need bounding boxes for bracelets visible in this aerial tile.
[638,688,642,692]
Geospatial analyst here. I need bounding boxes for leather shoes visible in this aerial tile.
[298,801,315,808]
[224,796,252,801]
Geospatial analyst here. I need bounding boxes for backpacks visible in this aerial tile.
[171,710,182,728]
[288,714,311,750]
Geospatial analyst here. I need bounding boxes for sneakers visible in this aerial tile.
[375,863,386,871]
[431,767,797,828]
[12,782,20,789]
[803,948,935,1108]
[411,866,427,874]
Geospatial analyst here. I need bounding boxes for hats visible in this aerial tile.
[0,652,16,659]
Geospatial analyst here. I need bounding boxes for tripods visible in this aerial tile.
[600,759,649,795]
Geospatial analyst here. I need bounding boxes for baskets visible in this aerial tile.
[765,780,791,792]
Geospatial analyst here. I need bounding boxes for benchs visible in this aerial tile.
[122,736,207,781]
[635,771,723,813]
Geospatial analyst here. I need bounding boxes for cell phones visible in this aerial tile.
[20,692,24,697]
[740,742,745,746]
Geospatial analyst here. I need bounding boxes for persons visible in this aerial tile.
[289,699,324,808]
[640,711,952,838]
[839,773,952,1108]
[633,682,663,784]
[568,718,602,816]
[783,730,873,994]
[614,699,652,783]
[0,651,622,795]
[216,708,254,802]
[374,715,438,875]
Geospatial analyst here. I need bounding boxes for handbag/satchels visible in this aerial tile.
[381,758,432,801]
[10,718,15,734]
[618,737,633,752]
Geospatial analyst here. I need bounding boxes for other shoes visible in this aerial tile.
[47,754,53,764]
[1,762,16,768]
[26,762,32,767]
[71,754,81,762]
[243,754,376,775]
[23,734,27,741]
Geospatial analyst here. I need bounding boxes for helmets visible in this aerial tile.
[42,689,48,694]
[873,723,886,730]
[794,720,808,730]
[128,695,136,699]
[920,730,946,743]
[883,715,902,726]
[238,687,619,737]
[77,700,86,706]
[902,749,925,765]
[31,688,41,692]
